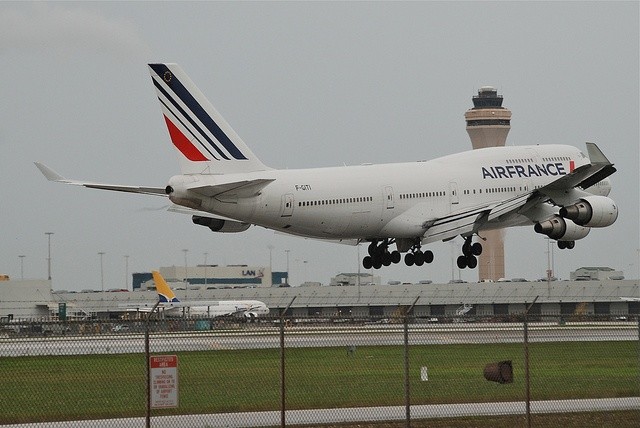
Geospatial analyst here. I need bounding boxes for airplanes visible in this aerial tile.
[32,62,619,269]
[115,270,271,324]
